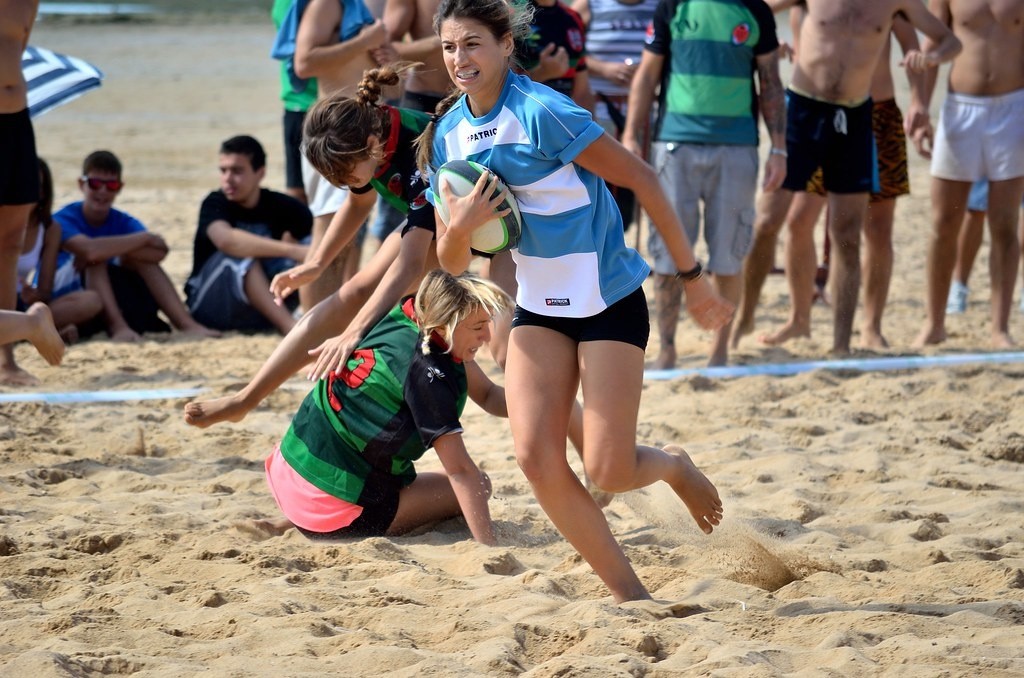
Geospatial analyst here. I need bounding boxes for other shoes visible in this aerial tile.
[945,281,972,314]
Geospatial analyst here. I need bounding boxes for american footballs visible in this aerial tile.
[435,159,523,254]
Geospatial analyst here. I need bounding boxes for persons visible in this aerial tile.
[183,135,316,337]
[266,268,517,574]
[423,3,735,600]
[272,0,1024,372]
[183,66,617,507]
[15,156,105,342]
[24,151,222,339]
[0,0,41,387]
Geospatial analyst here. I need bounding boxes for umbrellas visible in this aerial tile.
[20,46,104,121]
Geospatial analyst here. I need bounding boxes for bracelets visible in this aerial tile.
[675,262,704,285]
[770,148,788,158]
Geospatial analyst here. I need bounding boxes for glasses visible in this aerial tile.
[82,176,123,193]
